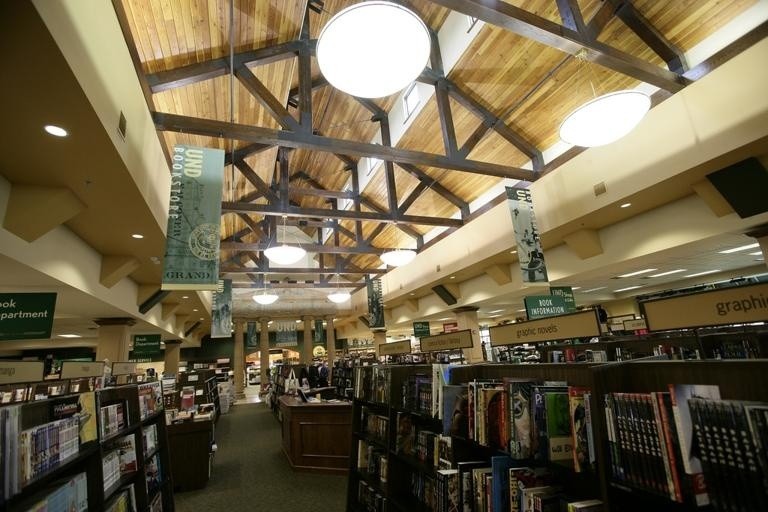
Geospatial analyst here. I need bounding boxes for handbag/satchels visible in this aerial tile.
[283,368,301,395]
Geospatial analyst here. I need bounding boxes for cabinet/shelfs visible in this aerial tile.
[260,355,354,424]
[346,325,768,511]
[0,356,225,512]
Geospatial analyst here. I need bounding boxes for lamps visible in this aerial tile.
[263,214,306,267]
[378,222,416,267]
[311,0,435,101]
[326,273,352,304]
[251,273,279,306]
[555,45,653,148]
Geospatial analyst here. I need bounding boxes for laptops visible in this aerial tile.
[297,389,321,403]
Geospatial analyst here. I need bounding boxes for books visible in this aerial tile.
[0,363,236,512]
